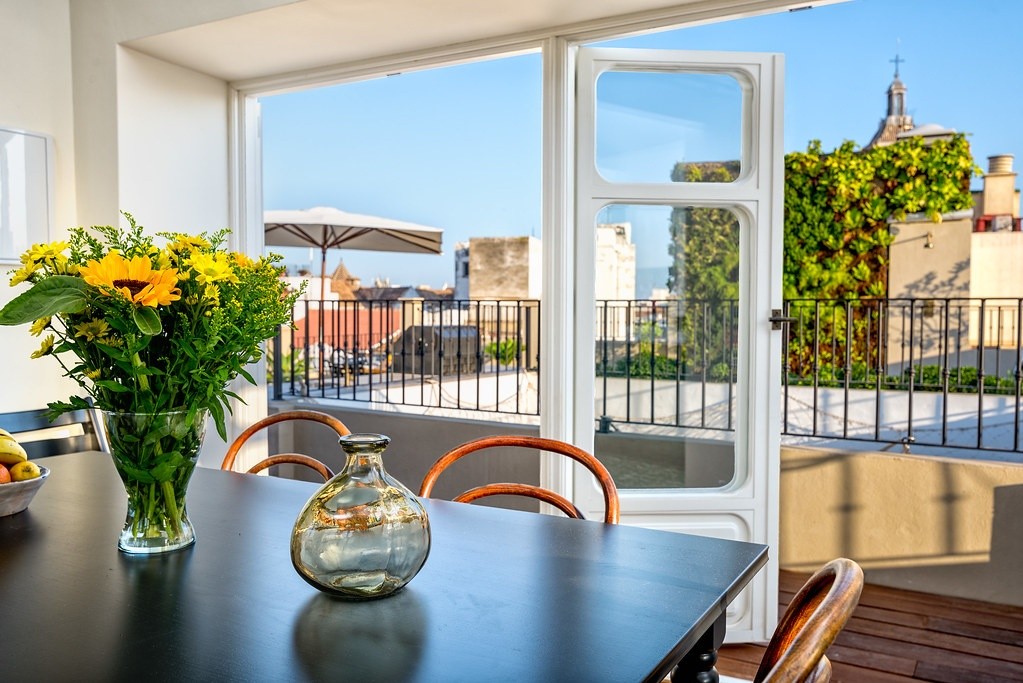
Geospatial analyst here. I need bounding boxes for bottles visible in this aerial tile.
[290,433,432,601]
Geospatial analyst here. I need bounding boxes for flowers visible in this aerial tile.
[0,208,309,543]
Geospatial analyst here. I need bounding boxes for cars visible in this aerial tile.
[338,349,388,375]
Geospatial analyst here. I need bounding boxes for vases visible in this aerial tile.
[290,432,433,598]
[100,407,209,554]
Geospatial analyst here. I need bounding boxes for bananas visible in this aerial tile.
[0,428,27,465]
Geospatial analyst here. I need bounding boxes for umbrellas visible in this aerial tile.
[264,207,442,390]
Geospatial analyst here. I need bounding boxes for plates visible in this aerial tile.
[0,464,52,517]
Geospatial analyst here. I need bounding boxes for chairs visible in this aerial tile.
[717,558,865,683]
[0,396,107,454]
[418,434,621,528]
[220,410,352,482]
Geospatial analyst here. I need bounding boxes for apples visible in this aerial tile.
[0,461,40,483]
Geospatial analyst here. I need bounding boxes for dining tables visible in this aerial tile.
[0,450,770,683]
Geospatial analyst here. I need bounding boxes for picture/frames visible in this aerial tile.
[1,128,54,266]
